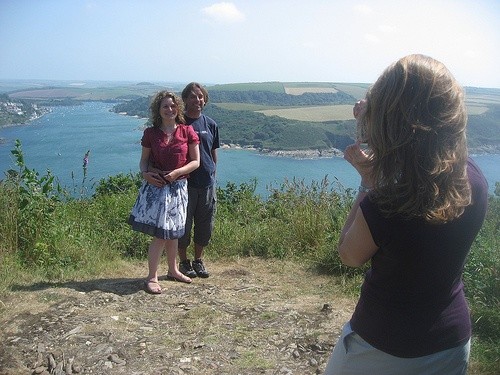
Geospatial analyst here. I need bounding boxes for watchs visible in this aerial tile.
[142,171,147,174]
[359,186,373,192]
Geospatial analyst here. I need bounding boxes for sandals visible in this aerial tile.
[167,270,192,283]
[144,278,162,293]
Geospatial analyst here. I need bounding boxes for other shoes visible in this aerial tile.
[179,260,196,277]
[192,260,209,277]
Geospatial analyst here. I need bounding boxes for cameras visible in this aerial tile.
[358,118,369,143]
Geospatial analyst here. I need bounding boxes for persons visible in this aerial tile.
[322,55,489,375]
[148,82,220,278]
[127,89,200,293]
